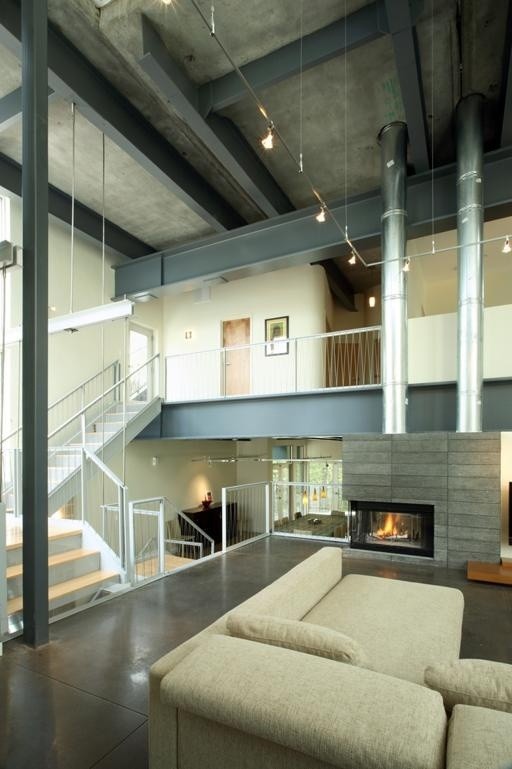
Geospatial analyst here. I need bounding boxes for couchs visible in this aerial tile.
[148,547,512,769]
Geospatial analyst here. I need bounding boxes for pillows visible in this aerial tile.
[423,658,512,712]
[226,612,365,666]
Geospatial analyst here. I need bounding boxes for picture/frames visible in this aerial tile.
[265,316,289,356]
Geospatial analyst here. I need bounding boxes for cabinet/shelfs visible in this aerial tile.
[179,503,237,552]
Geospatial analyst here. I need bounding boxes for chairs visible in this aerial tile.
[273,511,348,537]
[166,521,195,554]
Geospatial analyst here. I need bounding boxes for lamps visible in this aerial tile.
[303,461,326,504]
[260,122,511,273]
